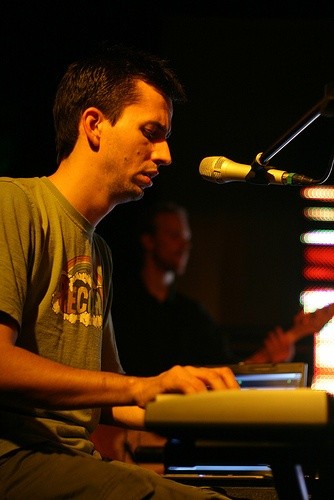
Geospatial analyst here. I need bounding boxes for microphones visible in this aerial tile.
[199,156,319,187]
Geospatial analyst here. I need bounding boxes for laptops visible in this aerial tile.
[161,363,308,481]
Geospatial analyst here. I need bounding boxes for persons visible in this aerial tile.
[0,52,242,500]
[111,201,296,428]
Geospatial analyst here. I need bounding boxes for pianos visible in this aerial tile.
[144,386,334,427]
